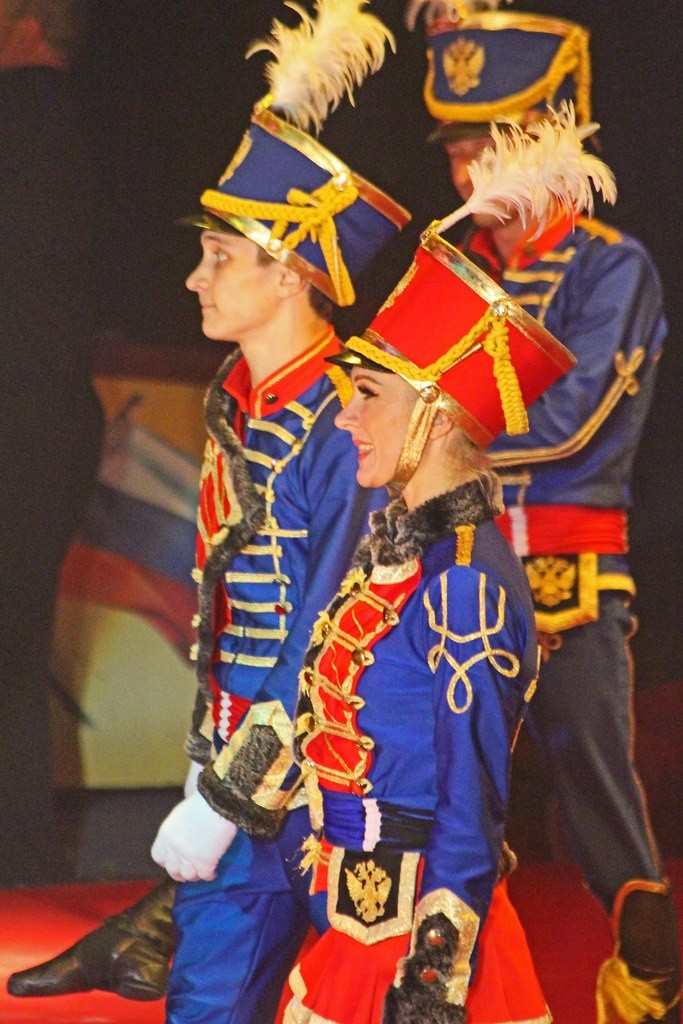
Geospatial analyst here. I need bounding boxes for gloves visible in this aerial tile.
[151,795,239,884]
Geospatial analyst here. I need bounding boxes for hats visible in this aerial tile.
[183,761,204,797]
[325,100,617,452]
[175,0,412,307]
[406,0,592,145]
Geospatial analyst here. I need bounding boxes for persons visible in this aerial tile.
[7,11,683,1024]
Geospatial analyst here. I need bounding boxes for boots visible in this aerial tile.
[596,876,683,1024]
[7,882,178,1001]
